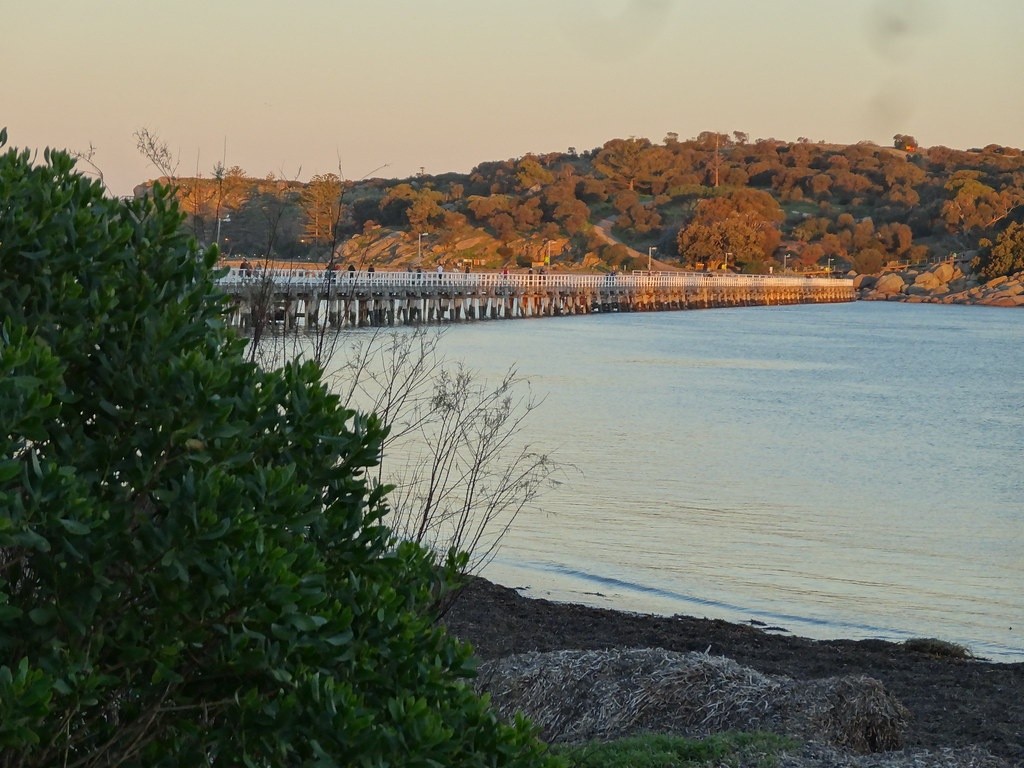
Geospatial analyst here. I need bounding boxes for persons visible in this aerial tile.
[502,267,510,279]
[437,263,444,279]
[540,267,545,274]
[368,263,376,278]
[601,271,616,286]
[529,266,533,274]
[239,257,262,286]
[417,266,421,276]
[453,266,459,272]
[408,263,412,272]
[465,264,470,273]
[348,265,356,278]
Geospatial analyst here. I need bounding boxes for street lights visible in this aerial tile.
[827,257,835,276]
[784,252,791,270]
[416,230,430,259]
[725,250,732,268]
[648,243,658,268]
[217,216,232,245]
[547,237,556,269]
